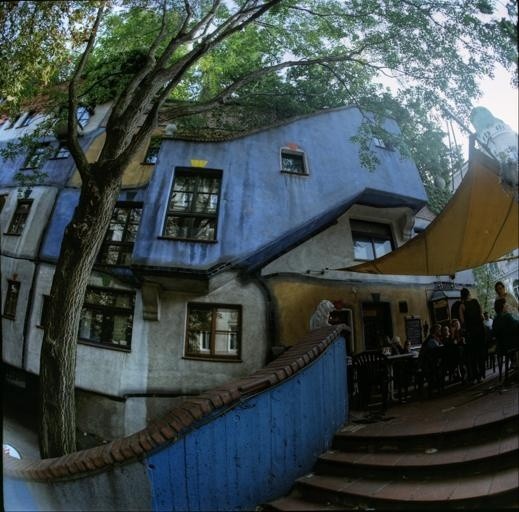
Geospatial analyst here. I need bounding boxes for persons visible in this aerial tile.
[394,279,519,401]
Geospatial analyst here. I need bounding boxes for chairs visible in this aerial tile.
[349,327,519,411]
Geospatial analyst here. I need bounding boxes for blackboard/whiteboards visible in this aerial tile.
[405,315,423,349]
